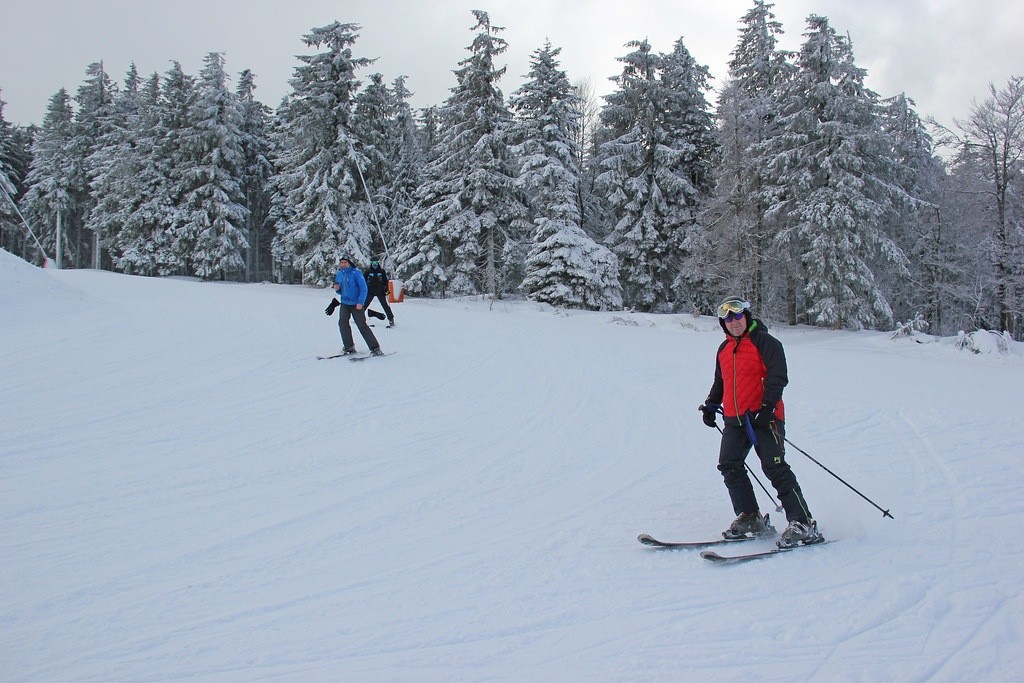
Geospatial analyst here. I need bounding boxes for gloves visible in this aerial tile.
[325,297,339,316]
[384,291,390,295]
[703,405,716,428]
[759,399,775,435]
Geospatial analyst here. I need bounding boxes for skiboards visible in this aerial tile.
[362,318,396,328]
[637,511,843,564]
[318,350,396,361]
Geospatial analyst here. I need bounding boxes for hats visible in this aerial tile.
[718,295,752,338]
[370,256,379,261]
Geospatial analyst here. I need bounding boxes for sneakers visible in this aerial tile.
[721,511,777,541]
[775,520,825,551]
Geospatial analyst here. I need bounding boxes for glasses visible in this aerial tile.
[342,256,349,259]
[725,313,744,322]
[717,301,751,319]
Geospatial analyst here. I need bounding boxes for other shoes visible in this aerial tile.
[373,347,384,356]
[389,319,394,326]
[344,345,355,354]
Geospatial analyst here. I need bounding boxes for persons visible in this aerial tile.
[333,253,384,358]
[362,256,395,326]
[702,296,825,549]
